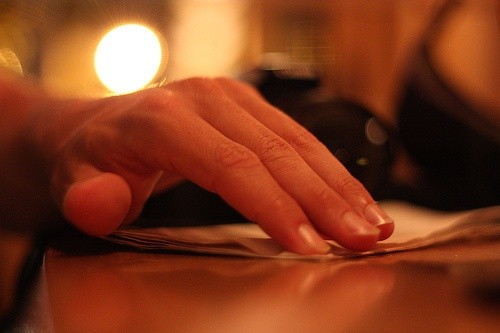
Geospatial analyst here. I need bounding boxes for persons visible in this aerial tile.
[338,0,500,207]
[0,73,398,258]
